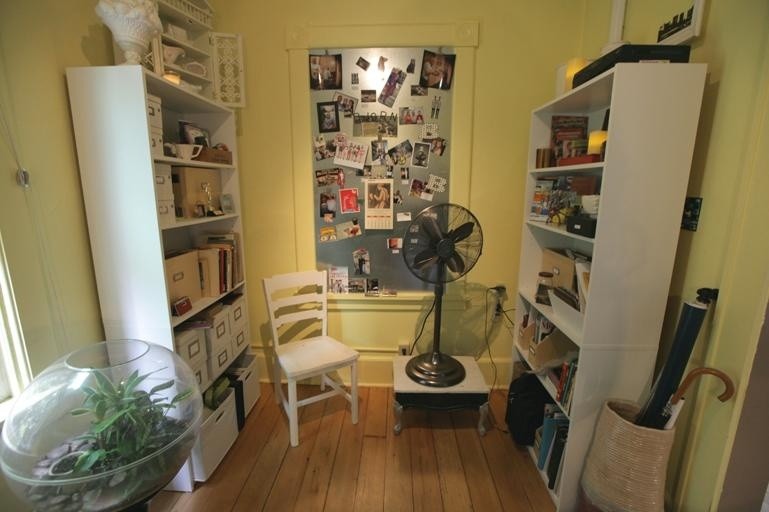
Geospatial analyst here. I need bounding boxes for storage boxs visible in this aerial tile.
[175,327,207,367]
[154,163,172,193]
[190,387,240,482]
[164,249,202,304]
[573,44,690,87]
[190,359,209,392]
[231,324,250,356]
[172,166,223,218]
[147,93,163,129]
[150,127,164,156]
[155,192,175,217]
[224,293,248,336]
[194,305,230,352]
[229,352,262,418]
[206,341,232,382]
[509,63,709,512]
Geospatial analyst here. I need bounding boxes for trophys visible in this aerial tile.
[199,182,226,217]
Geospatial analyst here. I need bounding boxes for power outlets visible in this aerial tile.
[491,297,503,321]
[399,342,410,356]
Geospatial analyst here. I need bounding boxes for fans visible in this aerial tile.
[402,202,484,387]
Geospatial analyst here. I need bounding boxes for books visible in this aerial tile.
[228,354,256,376]
[521,305,555,351]
[536,116,586,168]
[556,358,579,413]
[199,231,242,299]
[554,261,592,313]
[536,403,569,497]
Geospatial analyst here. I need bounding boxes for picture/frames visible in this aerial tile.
[610,0,705,45]
[316,101,340,133]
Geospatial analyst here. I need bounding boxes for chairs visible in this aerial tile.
[263,269,359,447]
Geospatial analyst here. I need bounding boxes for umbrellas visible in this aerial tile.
[654,367,735,430]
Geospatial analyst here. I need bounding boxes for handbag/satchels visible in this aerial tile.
[505,374,546,447]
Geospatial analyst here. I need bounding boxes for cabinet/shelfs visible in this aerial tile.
[66,63,262,492]
[113,0,246,109]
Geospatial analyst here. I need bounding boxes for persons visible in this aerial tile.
[405,112,413,124]
[431,95,442,119]
[421,56,439,87]
[374,185,389,209]
[337,142,366,163]
[335,280,344,292]
[431,139,444,155]
[417,110,426,124]
[356,255,366,274]
[335,95,354,112]
